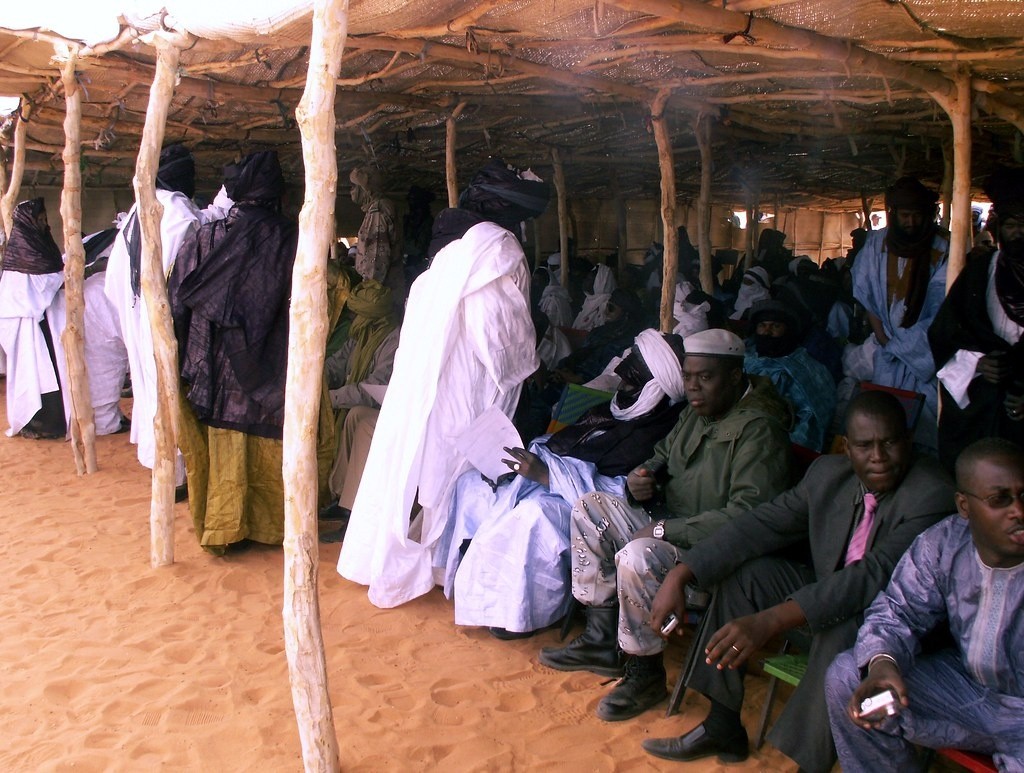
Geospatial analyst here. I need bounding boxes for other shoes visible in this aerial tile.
[175,483,187,503]
[490,617,563,639]
[24,414,66,437]
[109,416,131,433]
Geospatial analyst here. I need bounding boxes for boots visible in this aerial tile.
[539,606,623,681]
[597,652,668,720]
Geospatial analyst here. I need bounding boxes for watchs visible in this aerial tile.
[653,519,666,540]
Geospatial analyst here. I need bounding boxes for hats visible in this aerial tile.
[683,329,747,359]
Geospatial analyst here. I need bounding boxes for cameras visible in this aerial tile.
[858,689,905,725]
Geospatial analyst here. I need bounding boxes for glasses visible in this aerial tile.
[958,488,1024,508]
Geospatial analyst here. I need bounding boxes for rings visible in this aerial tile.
[731,645,740,652]
[514,464,520,471]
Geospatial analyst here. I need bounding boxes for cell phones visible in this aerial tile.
[659,612,678,636]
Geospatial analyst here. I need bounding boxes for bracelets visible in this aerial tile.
[867,652,901,672]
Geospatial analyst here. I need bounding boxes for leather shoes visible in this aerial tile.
[319,521,349,544]
[319,501,350,520]
[642,721,750,762]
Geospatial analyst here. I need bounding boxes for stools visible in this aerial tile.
[540,383,999,773]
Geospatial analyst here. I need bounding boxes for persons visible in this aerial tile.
[775,177,1024,459]
[642,391,957,773]
[0,137,789,772]
[820,434,1024,772]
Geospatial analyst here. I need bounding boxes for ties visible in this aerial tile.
[845,493,876,567]
